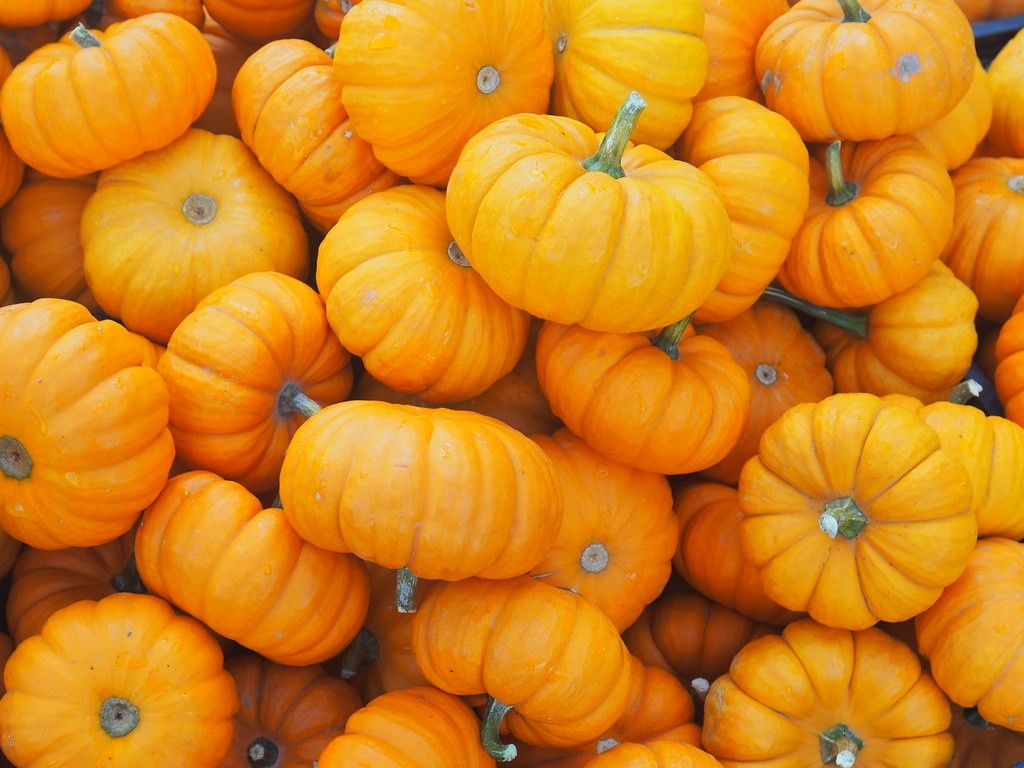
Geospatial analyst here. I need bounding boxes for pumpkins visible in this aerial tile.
[0,0,1023,768]
